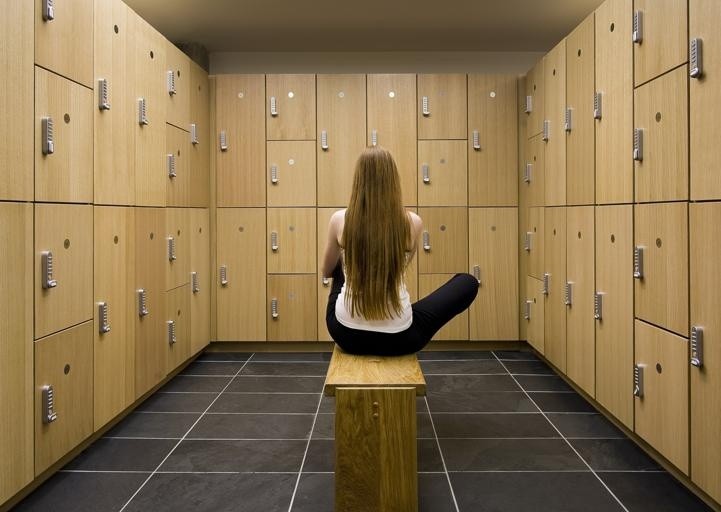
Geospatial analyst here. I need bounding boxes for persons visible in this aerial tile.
[320,143,479,358]
